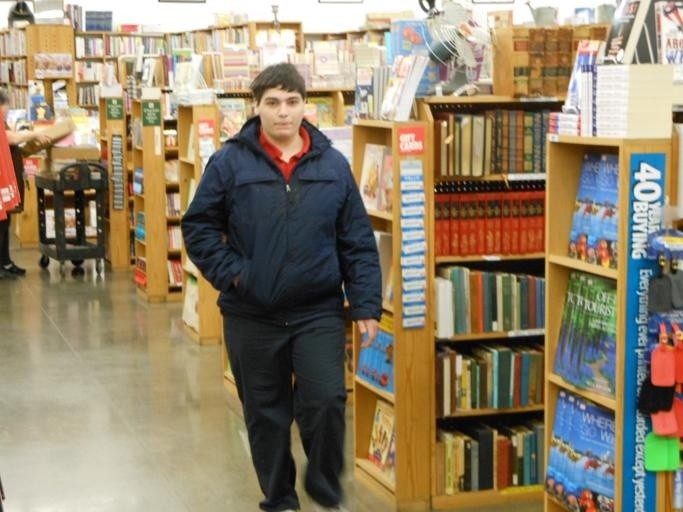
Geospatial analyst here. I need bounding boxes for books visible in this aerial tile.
[255,29,388,163]
[0,1,287,331]
[354,0,683,511]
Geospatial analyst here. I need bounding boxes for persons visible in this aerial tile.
[180,63,382,512]
[0,95,53,281]
[372,425,389,462]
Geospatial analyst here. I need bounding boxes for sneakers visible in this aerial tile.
[5,265,25,274]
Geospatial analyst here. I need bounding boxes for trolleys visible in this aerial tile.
[32,159,108,276]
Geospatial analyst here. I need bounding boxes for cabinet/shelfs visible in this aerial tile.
[166,22,303,88]
[99,97,135,274]
[353,93,567,511]
[75,30,167,115]
[0,24,77,110]
[305,28,390,45]
[180,87,353,346]
[543,120,683,512]
[487,10,609,93]
[130,99,180,304]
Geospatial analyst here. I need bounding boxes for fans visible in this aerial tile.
[417,0,495,96]
